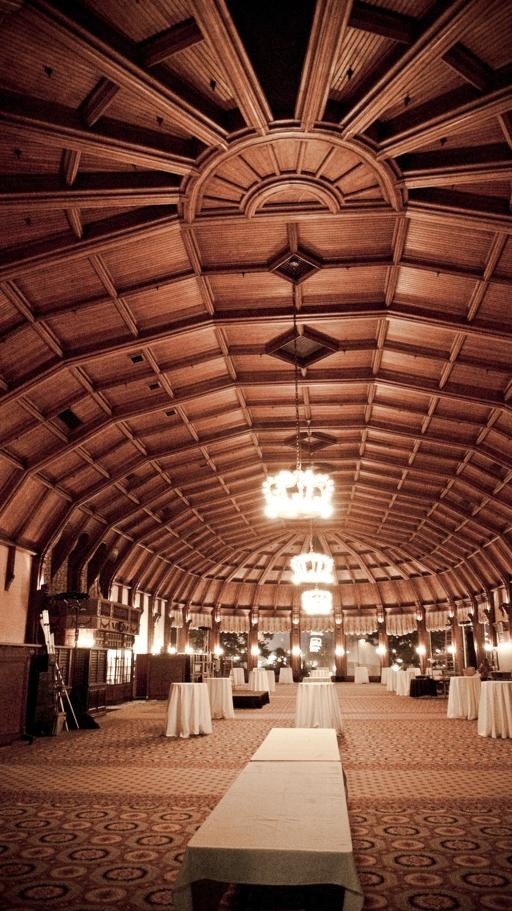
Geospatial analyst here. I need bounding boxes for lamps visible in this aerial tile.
[260,253,340,618]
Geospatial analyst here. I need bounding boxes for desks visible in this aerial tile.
[380,667,512,741]
[295,666,370,738]
[168,666,293,739]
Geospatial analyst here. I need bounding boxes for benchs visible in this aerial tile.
[185,728,355,911]
